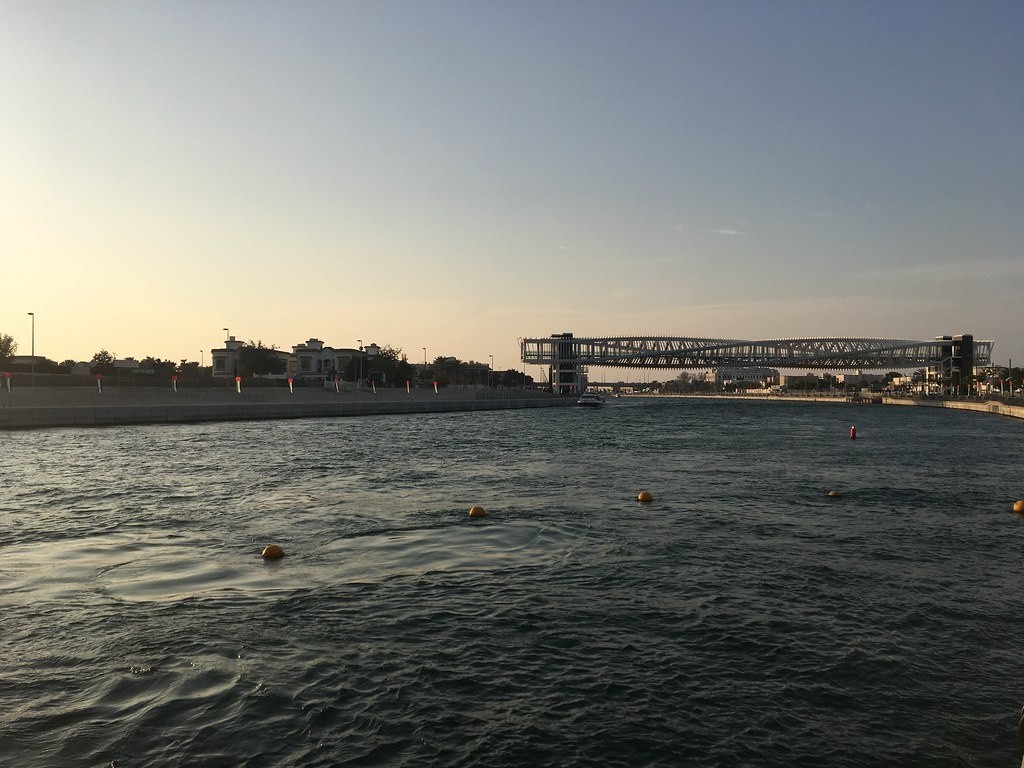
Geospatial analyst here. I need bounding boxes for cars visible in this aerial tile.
[984,393,1000,400]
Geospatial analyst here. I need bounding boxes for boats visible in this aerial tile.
[574,393,605,410]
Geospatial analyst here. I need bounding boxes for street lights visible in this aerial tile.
[28,313,35,386]
[423,348,426,364]
[200,350,203,367]
[223,328,229,341]
[357,340,362,385]
[490,355,493,372]
